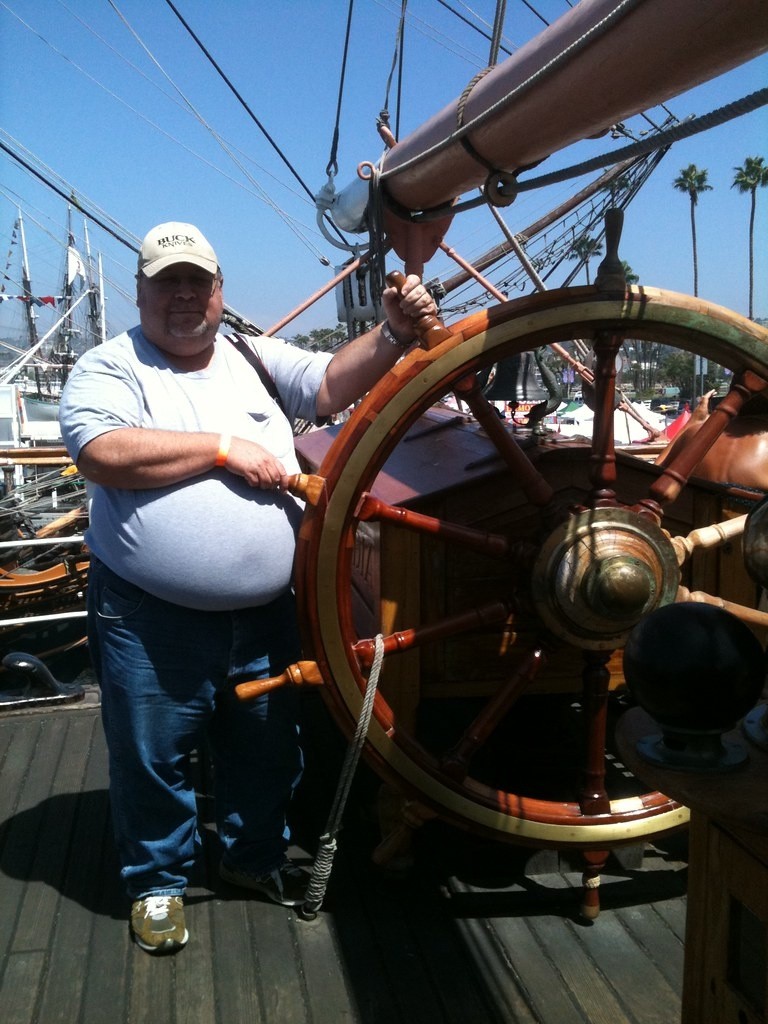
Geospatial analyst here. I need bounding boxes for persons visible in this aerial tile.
[59,222,440,955]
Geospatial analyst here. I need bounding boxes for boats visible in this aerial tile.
[543,392,674,444]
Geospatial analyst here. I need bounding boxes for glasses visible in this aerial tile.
[149,273,220,292]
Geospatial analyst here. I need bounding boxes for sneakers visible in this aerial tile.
[220,851,313,906]
[130,895,188,951]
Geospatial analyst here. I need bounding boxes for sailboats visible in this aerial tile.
[0,192,111,420]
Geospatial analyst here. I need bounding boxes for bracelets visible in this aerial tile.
[381,319,417,348]
[215,433,231,467]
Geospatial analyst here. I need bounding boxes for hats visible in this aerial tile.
[138,222,221,278]
